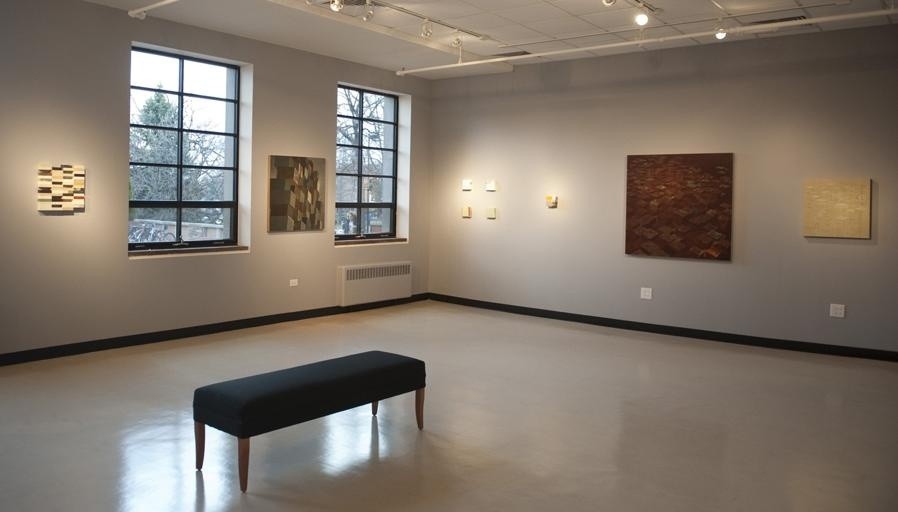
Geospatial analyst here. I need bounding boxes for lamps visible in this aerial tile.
[330,1,481,49]
[715,7,726,42]
[602,1,656,29]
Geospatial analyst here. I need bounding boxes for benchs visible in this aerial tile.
[193,350,426,493]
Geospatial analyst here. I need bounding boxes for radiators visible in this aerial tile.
[340,261,412,306]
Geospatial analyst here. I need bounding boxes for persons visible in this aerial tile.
[341,211,357,235]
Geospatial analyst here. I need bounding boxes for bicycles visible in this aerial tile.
[128,222,175,241]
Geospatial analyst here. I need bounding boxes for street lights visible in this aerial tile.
[366,132,378,234]
